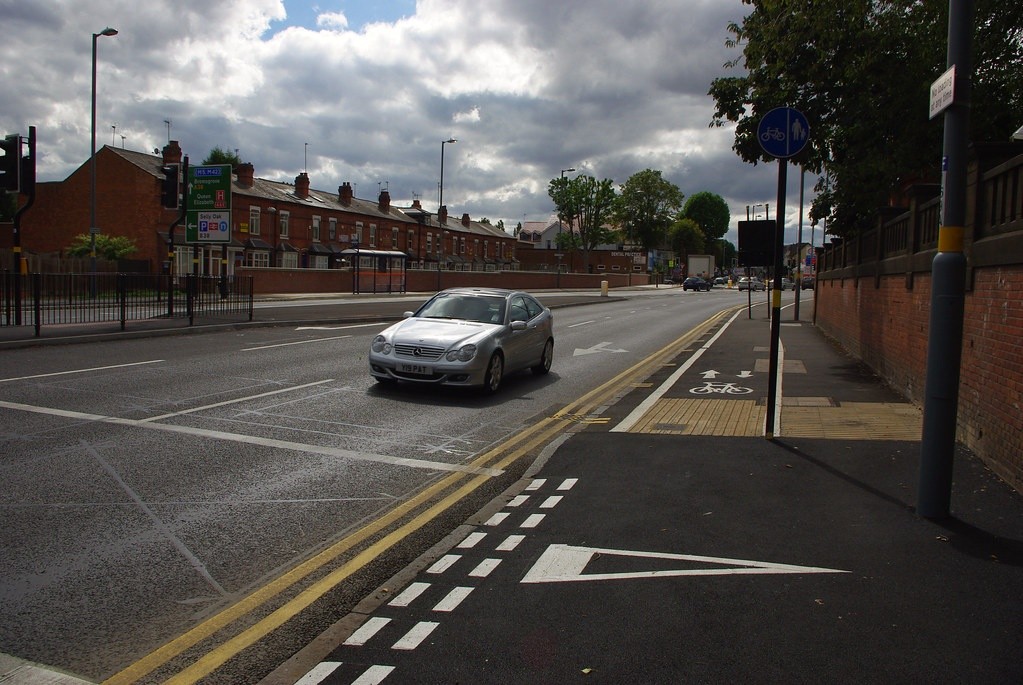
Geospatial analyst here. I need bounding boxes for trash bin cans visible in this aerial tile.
[601,281,608,297]
[728,280,732,288]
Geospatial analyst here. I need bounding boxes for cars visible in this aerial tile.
[368,287,555,397]
[739,277,766,291]
[769,278,796,291]
[715,277,724,285]
[683,277,711,291]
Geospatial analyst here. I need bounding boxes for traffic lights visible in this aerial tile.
[0,134,22,194]
[162,163,180,210]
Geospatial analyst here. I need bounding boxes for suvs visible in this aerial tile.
[802,277,815,290]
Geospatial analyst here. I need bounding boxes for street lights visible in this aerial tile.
[91,27,118,297]
[437,138,458,291]
[305,143,308,173]
[112,126,115,147]
[557,168,575,288]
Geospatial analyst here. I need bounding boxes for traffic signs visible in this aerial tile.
[184,164,233,245]
